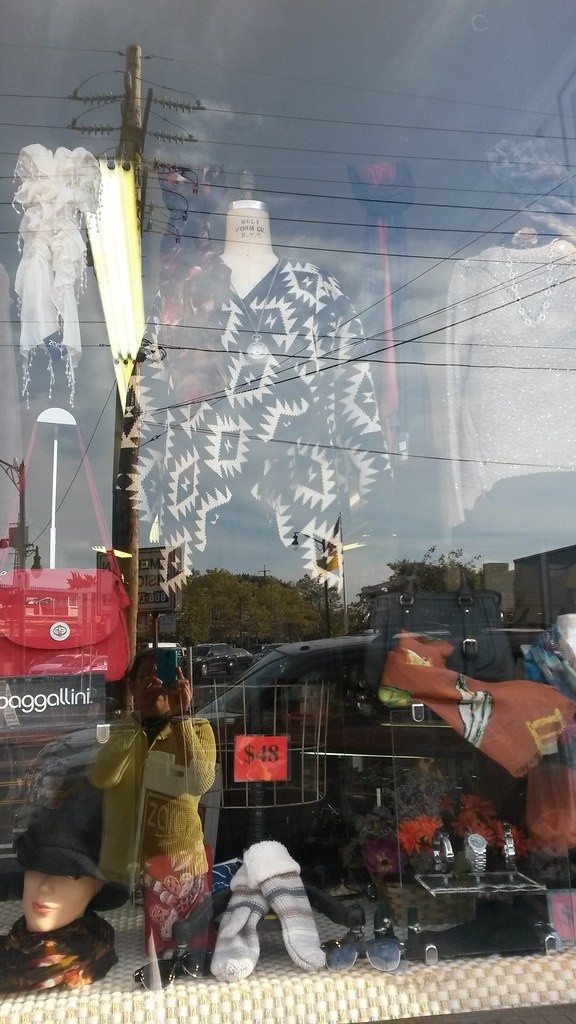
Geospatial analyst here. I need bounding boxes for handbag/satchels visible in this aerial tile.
[360,549,525,704]
[0,418,130,683]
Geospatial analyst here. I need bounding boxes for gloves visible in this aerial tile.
[210,841,326,982]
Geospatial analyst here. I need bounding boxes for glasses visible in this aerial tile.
[323,938,406,972]
[132,951,216,992]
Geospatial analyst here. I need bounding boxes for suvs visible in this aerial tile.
[10,622,575,887]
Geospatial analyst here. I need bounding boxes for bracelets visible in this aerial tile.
[502,822,517,872]
[433,829,454,874]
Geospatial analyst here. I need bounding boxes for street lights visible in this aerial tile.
[291,531,330,638]
[36,408,77,571]
[19,546,42,677]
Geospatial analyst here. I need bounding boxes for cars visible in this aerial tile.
[27,654,76,675]
[137,642,184,658]
[180,643,237,677]
[42,654,108,675]
[249,643,289,667]
[233,647,253,664]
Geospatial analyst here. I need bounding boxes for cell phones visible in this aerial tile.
[156,647,177,688]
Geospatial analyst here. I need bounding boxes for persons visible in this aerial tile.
[87,650,216,888]
[117,201,393,611]
[0,808,127,995]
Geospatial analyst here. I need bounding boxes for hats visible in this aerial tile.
[14,778,131,912]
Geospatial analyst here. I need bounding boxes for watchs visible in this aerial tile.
[464,832,488,874]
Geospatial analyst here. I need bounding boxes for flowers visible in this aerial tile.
[362,762,527,876]
